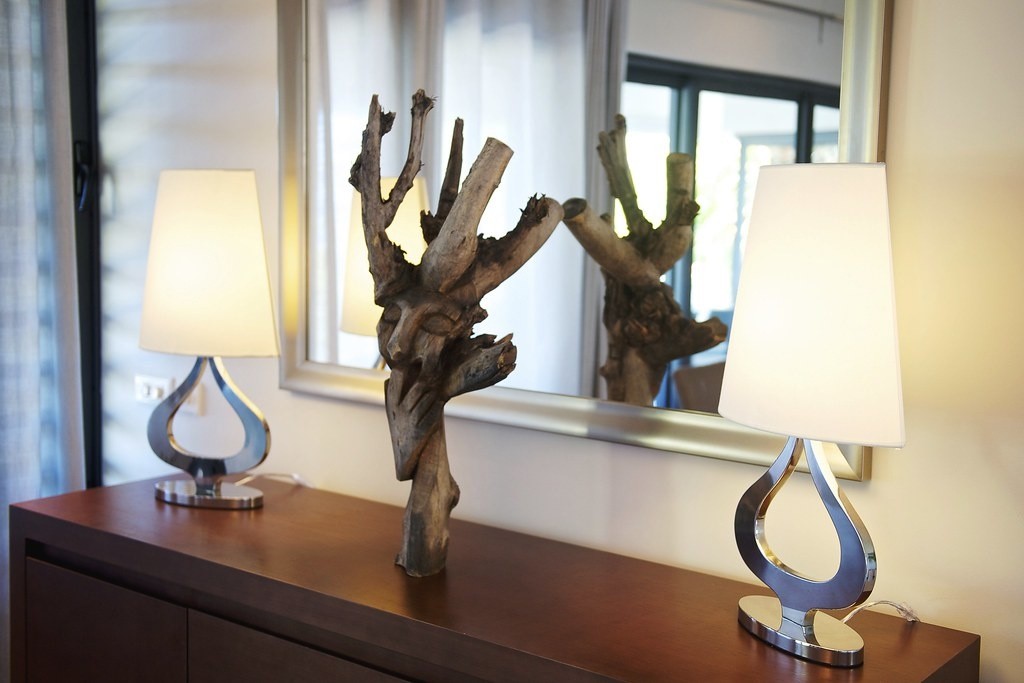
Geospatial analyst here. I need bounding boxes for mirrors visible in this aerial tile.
[275,0,894,483]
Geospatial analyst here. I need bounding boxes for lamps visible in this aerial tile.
[717,162,905,668]
[341,178,431,369]
[140,168,280,510]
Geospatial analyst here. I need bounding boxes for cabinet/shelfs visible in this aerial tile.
[9,475,982,683]
